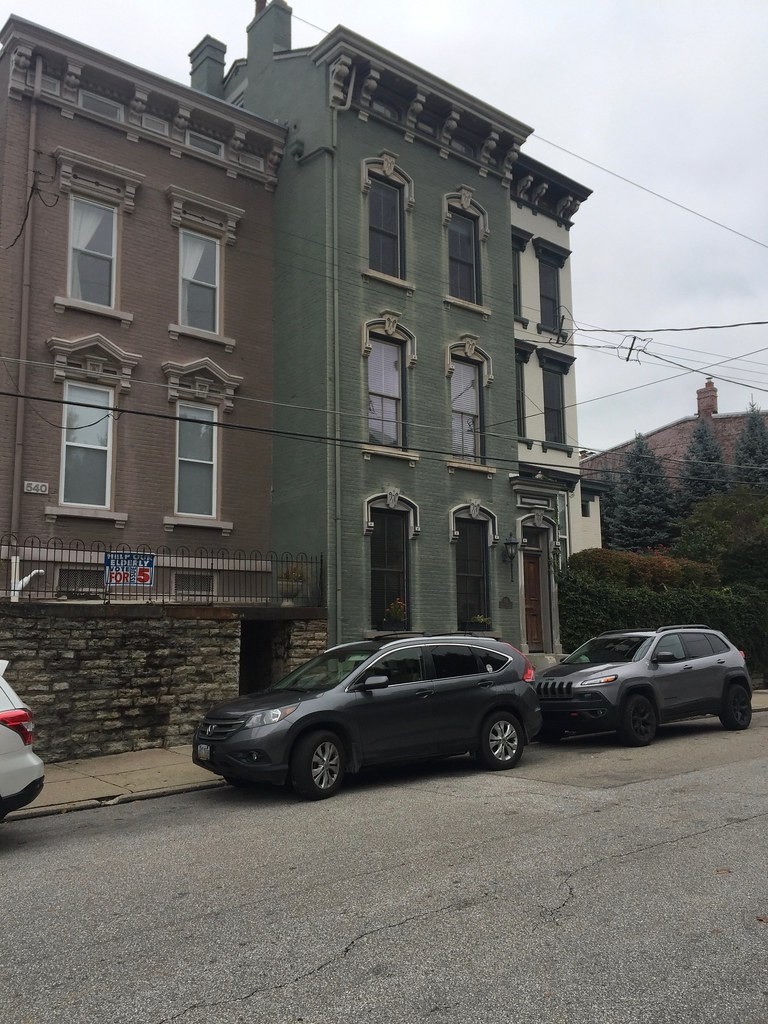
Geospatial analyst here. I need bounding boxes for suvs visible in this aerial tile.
[533,625,754,748]
[192,631,543,802]
[0,660,47,822]
